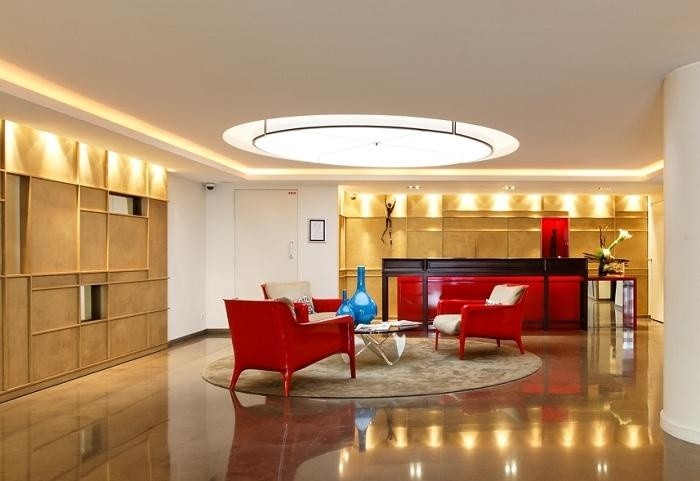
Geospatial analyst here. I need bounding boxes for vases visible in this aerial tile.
[347,266,377,325]
[336,289,355,324]
[598,257,625,277]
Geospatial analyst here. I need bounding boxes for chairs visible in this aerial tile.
[224,297,356,395]
[261,281,343,311]
[224,390,355,481]
[433,282,530,359]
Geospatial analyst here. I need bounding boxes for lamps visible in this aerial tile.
[222,114,521,168]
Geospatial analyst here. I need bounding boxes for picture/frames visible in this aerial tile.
[310,220,325,241]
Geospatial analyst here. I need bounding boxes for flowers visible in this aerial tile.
[583,225,632,262]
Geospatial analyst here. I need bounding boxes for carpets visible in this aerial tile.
[201,336,542,398]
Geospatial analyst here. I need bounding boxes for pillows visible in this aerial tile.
[294,296,316,314]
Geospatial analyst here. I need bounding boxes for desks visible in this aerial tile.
[587,275,641,331]
